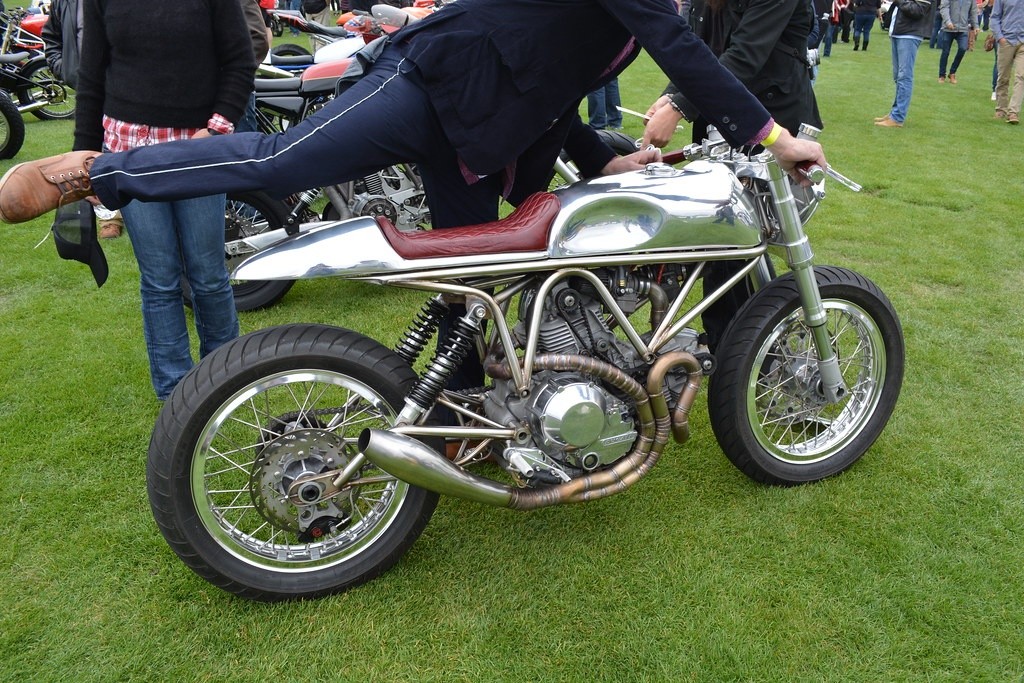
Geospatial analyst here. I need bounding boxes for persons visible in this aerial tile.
[71,1,255,403]
[44,0,1024,239]
[1,0,825,469]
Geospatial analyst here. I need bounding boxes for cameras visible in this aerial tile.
[806,46,821,80]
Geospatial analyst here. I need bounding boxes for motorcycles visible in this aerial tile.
[0,0,645,319]
[146,116,905,603]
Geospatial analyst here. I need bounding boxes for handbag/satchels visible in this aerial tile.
[984,34,995,52]
[966,30,976,51]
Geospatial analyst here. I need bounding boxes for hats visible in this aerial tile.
[51,199,109,288]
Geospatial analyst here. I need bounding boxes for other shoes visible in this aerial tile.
[100,223,122,237]
[854,41,859,50]
[285,29,292,33]
[991,91,997,101]
[938,75,946,84]
[446,439,499,463]
[293,31,299,37]
[948,73,957,84]
[994,110,1006,119]
[876,119,903,128]
[0,150,105,225]
[861,42,868,50]
[1005,115,1020,123]
[873,112,893,122]
